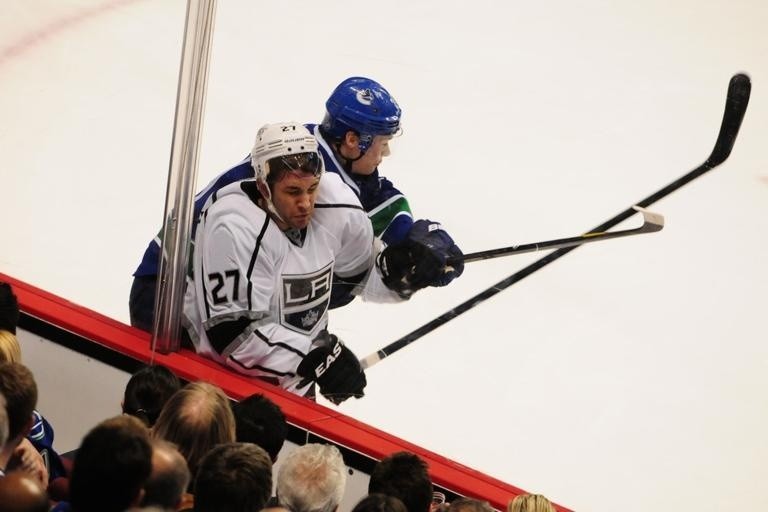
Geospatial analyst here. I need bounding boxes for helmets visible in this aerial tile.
[320,77,402,153]
[251,122,325,221]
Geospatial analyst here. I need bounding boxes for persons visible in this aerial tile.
[179,121,448,407]
[0,282,557,512]
[128,74,464,333]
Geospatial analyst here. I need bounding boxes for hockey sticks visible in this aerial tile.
[445,203,664,265]
[357,74,752,371]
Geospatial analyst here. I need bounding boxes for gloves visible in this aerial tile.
[297,330,367,404]
[380,219,464,299]
